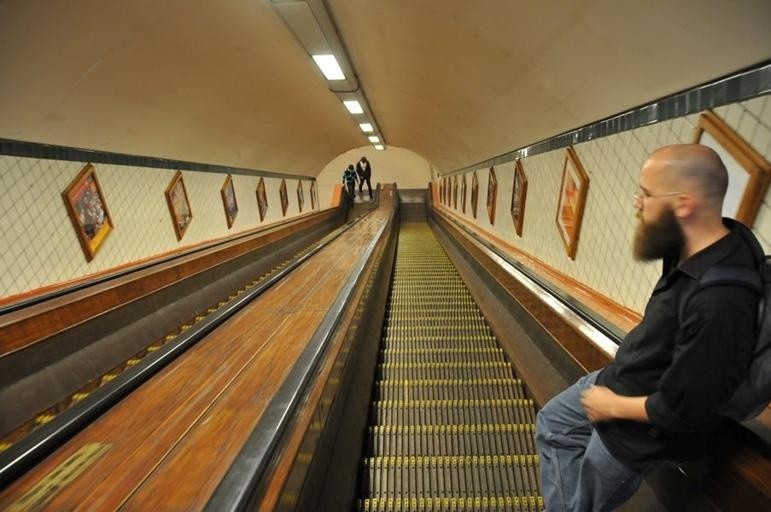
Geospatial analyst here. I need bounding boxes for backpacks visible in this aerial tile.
[678,254,770,420]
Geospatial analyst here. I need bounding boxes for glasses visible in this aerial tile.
[630,188,682,203]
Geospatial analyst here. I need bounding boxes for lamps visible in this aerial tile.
[268,1,388,152]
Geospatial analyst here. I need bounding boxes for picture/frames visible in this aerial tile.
[60,161,115,262]
[220,173,317,230]
[554,144,591,262]
[163,170,194,242]
[689,109,771,229]
[438,160,528,237]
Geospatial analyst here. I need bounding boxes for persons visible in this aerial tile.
[357,156,375,203]
[527,140,770,512]
[341,164,360,199]
